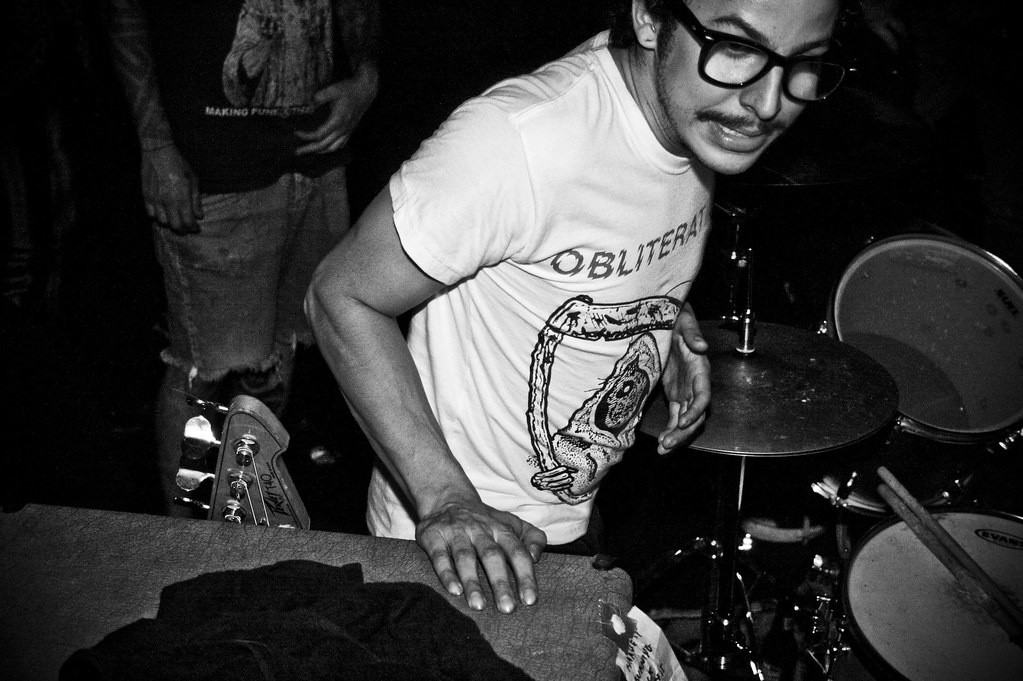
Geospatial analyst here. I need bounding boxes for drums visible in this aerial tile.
[791,230,1023,512]
[824,512,1023,681]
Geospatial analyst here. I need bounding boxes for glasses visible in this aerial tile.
[663,0,856,105]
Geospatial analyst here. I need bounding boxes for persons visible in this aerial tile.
[105,1,391,522]
[304,0,858,612]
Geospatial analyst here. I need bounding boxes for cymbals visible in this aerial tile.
[634,320,898,458]
[696,83,934,188]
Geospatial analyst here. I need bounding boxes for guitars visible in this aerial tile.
[177,364,315,527]
